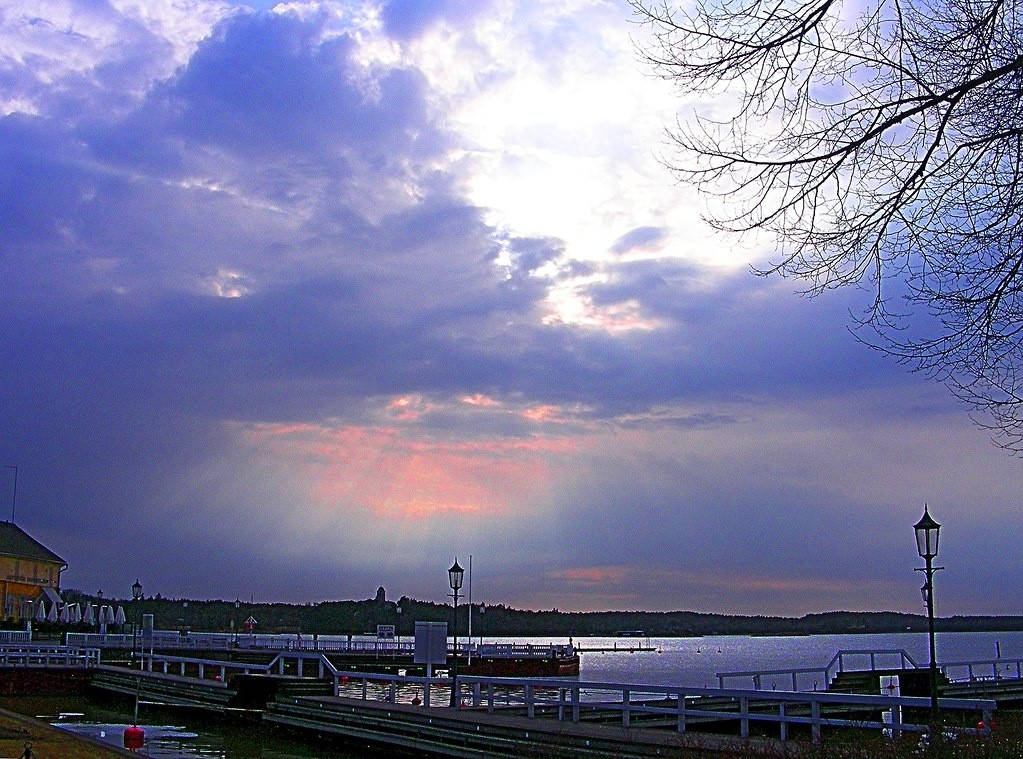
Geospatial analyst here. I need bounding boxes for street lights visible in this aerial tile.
[182,599,188,637]
[396,603,402,649]
[446,555,464,707]
[478,600,485,646]
[97,587,103,633]
[129,578,142,671]
[914,502,945,743]
[234,597,239,648]
